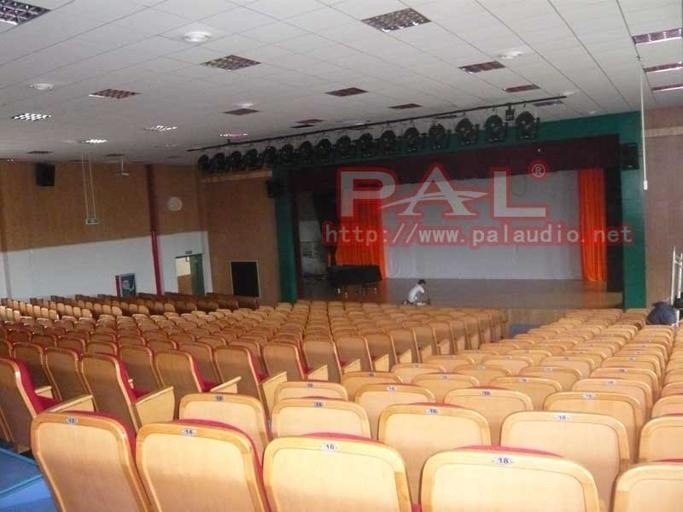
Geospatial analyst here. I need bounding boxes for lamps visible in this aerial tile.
[194,99,539,181]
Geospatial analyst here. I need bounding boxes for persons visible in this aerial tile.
[643,296,682,325]
[403,279,426,306]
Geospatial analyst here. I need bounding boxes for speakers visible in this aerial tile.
[620,142,640,170]
[267,182,279,196]
[36,162,56,186]
